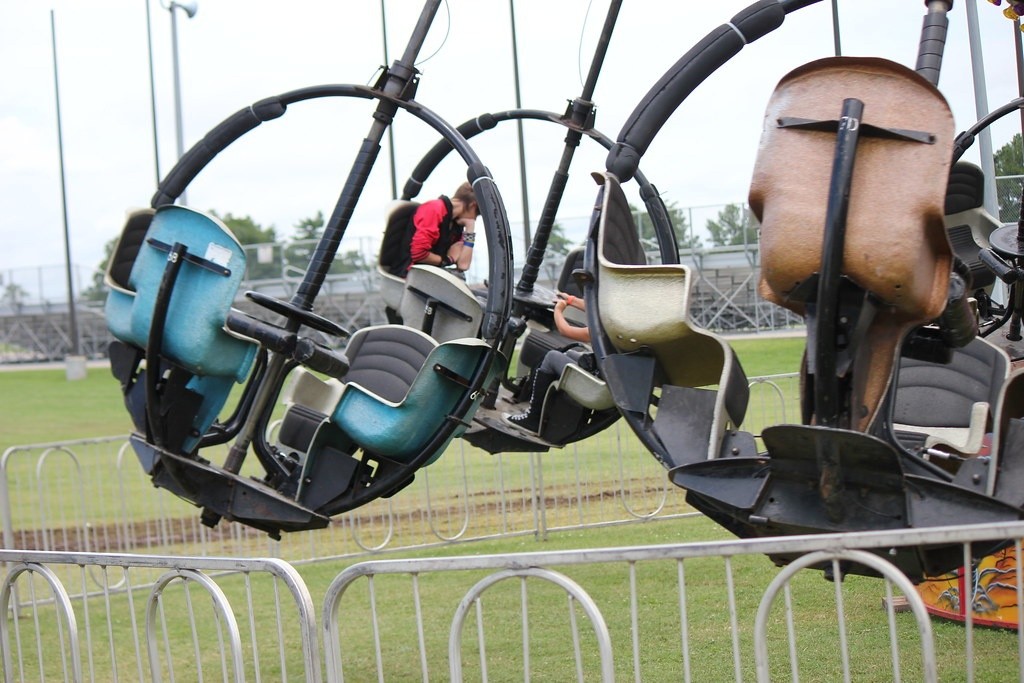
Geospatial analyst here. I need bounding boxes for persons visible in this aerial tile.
[385,183,481,334]
[504,292,601,436]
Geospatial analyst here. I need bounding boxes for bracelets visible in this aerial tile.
[463,242,474,247]
[567,295,575,305]
[437,254,454,268]
[462,232,476,241]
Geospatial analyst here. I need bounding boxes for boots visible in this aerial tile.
[501,369,555,435]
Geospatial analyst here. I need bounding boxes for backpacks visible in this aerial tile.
[379,200,421,276]
